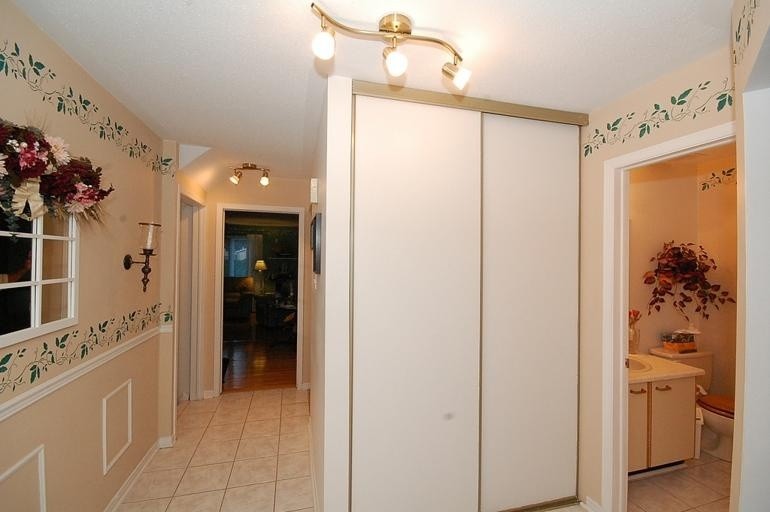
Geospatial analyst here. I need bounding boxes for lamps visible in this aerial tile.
[312,3,472,90]
[230,164,270,186]
[254,259,268,295]
[123,222,161,293]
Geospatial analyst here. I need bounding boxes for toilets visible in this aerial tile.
[650,337,733,462]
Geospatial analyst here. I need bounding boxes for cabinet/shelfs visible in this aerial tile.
[349,78,589,512]
[628,376,696,480]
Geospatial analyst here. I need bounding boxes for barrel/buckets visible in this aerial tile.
[284,274,297,305]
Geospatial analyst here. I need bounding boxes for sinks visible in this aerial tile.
[629,354,653,375]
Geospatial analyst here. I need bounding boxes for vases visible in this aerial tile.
[629,325,641,355]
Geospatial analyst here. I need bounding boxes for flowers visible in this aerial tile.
[0,119,116,244]
[629,309,643,326]
[641,240,737,334]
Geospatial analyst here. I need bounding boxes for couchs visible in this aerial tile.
[224,292,252,323]
